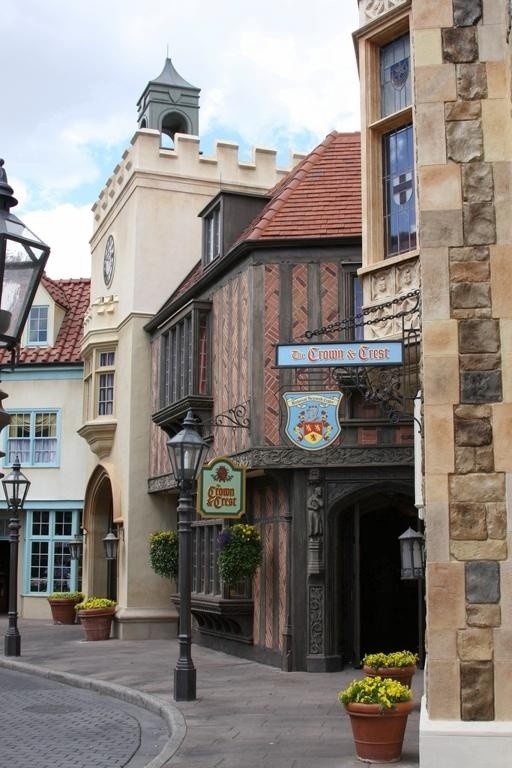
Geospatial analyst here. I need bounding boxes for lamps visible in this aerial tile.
[66,533,87,561]
[100,525,125,561]
[399,529,426,580]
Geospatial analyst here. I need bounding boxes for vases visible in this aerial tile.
[50,601,115,641]
[344,704,414,762]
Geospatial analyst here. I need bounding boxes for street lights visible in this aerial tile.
[167,413,208,702]
[2,457,30,654]
[0,160,48,481]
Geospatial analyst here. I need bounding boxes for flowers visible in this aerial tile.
[48,592,119,612]
[148,529,180,552]
[339,649,422,711]
[217,524,261,546]
